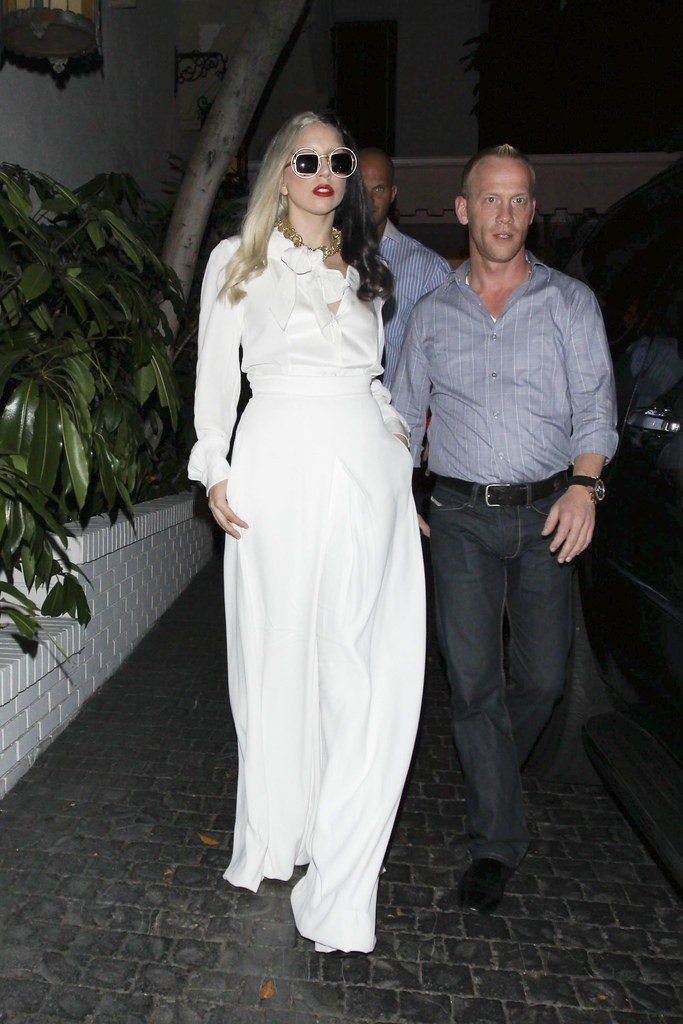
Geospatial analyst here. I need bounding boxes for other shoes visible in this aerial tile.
[334,949,360,958]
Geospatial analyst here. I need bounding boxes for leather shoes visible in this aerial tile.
[457,858,512,916]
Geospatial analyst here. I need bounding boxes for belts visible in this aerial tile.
[435,465,573,508]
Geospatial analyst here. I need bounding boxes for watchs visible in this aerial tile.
[566,476,605,504]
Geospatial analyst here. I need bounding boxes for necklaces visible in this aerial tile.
[276,216,342,260]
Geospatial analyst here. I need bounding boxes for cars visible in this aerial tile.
[558,156,683,903]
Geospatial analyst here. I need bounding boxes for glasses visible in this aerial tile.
[284,147,358,179]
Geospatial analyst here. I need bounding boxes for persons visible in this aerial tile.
[358,147,453,390]
[187,112,428,953]
[391,143,619,915]
